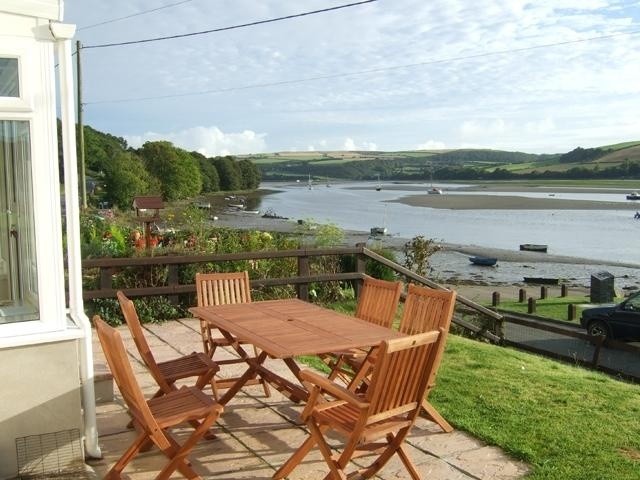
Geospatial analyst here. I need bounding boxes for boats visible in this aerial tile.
[469,256,498,266]
[626,192,640,200]
[306,173,313,190]
[427,187,442,195]
[375,176,383,191]
[519,243,549,251]
[371,227,387,233]
[326,175,333,187]
[243,210,260,214]
[228,202,244,209]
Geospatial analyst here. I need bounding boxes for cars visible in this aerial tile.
[576,288,639,347]
